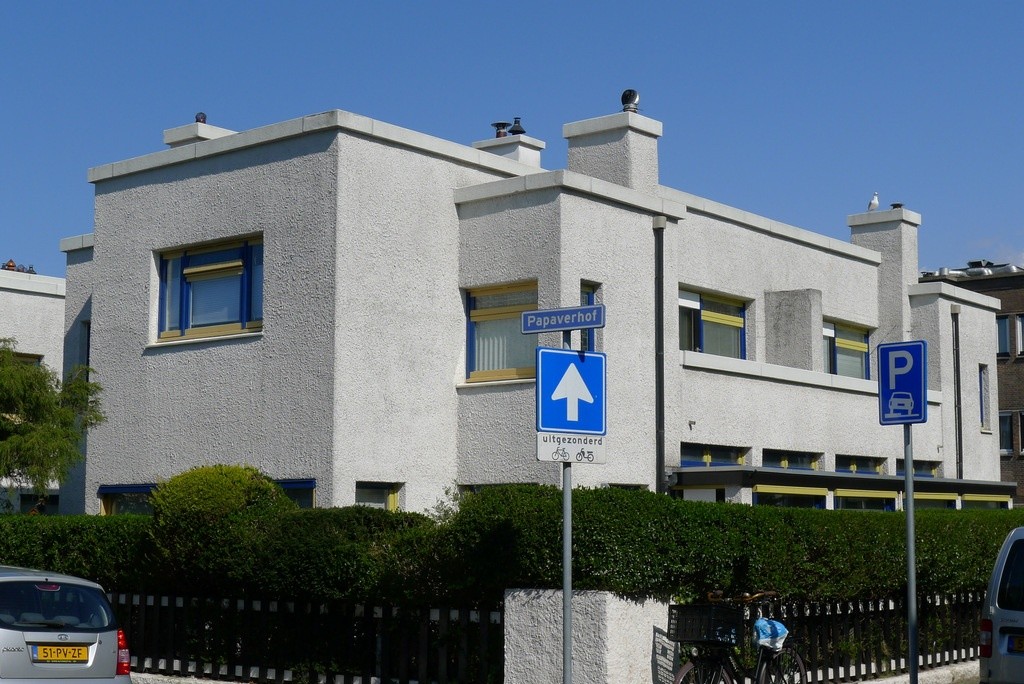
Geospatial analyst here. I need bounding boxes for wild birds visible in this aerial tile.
[867,191,879,212]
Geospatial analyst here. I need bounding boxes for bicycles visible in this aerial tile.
[667,588,807,684]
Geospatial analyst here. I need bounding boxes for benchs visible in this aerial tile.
[0,600,89,628]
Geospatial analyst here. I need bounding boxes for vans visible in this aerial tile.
[980,526,1024,684]
[0,564,133,683]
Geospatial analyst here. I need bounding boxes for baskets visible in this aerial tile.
[666,600,744,648]
